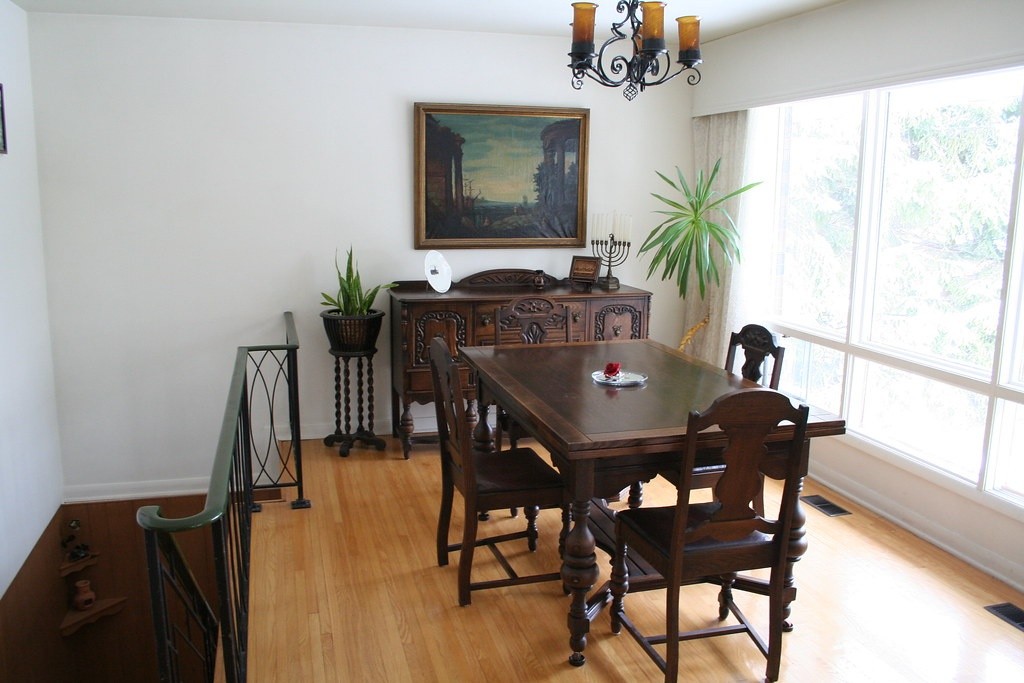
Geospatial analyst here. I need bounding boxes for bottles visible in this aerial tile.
[71,579,96,609]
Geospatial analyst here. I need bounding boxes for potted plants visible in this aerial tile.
[319,242,399,351]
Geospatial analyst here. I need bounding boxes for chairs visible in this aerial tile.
[427,336,572,607]
[608,388,811,683]
[658,323,786,517]
[494,294,574,517]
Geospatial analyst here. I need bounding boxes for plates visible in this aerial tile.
[592,370,645,386]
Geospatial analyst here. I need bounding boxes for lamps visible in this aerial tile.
[566,0,703,101]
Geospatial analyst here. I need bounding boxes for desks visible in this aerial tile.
[323,347,386,457]
[456,337,847,666]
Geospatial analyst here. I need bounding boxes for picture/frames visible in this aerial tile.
[569,256,603,286]
[412,102,591,249]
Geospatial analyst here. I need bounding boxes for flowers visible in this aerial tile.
[603,361,621,380]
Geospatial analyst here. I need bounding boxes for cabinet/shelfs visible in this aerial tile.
[60,551,128,636]
[387,268,653,460]
